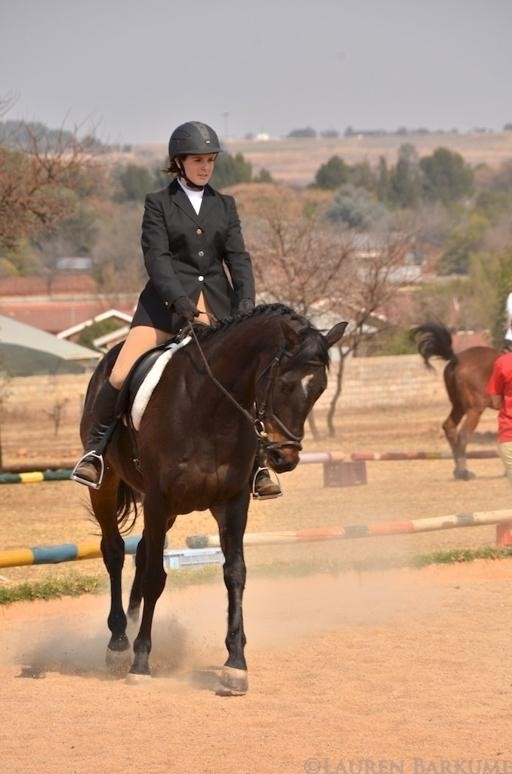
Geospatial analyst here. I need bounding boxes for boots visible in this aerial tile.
[72,377,129,483]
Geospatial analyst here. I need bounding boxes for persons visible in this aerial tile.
[76,119,279,497]
[487,325,512,479]
[503,289,512,349]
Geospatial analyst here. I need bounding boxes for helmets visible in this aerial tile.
[168,121,223,161]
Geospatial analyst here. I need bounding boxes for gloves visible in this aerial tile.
[236,296,255,317]
[170,295,201,323]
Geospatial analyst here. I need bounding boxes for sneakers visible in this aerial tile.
[253,474,283,497]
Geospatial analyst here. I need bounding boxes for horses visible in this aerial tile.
[77,301,349,697]
[408,321,512,482]
